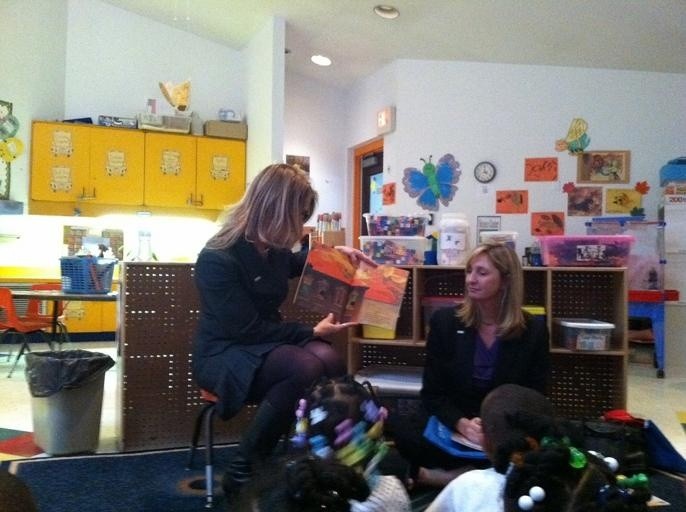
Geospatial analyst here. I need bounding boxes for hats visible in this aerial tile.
[480,384,553,473]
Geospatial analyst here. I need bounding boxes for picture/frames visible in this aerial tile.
[576,150,629,183]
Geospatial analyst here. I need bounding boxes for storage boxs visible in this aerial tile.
[553,317,615,352]
[536,235,635,267]
[357,210,431,267]
[420,297,464,341]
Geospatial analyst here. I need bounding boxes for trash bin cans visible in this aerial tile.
[24,348,109,455]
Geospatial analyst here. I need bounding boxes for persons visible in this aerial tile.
[190,163,348,510]
[427,383,549,510]
[289,370,412,512]
[416,239,549,489]
[490,430,651,510]
[221,458,371,511]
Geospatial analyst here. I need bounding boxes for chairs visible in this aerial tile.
[0,283,73,378]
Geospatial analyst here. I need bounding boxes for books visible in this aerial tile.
[292,239,412,337]
[448,429,487,453]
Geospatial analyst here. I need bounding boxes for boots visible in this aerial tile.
[222,399,292,496]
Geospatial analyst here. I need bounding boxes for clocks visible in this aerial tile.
[473,161,495,183]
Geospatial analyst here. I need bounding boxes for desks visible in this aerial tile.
[9,290,117,353]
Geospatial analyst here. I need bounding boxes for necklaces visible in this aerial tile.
[479,320,496,328]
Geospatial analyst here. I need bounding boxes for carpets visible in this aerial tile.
[0,417,685,512]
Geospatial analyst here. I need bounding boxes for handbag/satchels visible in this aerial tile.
[578,421,646,473]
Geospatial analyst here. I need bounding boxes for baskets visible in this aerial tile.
[60,256,114,293]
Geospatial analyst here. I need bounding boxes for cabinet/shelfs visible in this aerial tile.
[29,120,246,212]
[346,266,628,420]
